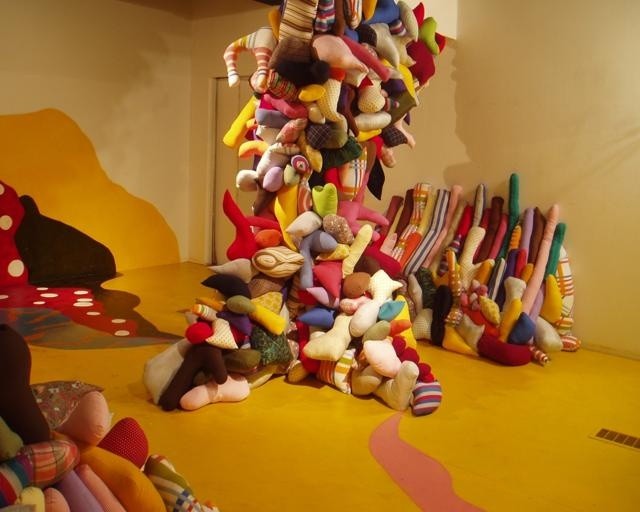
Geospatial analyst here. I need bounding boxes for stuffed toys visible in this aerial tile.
[0,0,582,511]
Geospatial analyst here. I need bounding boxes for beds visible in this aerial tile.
[3,112,639,510]
